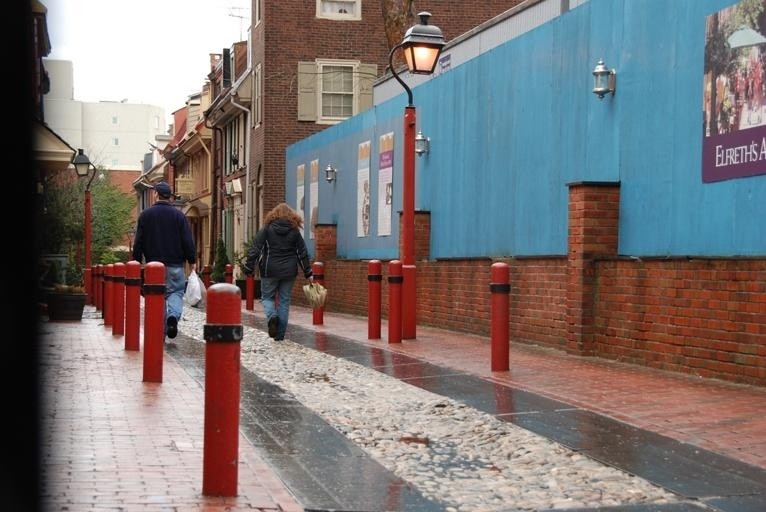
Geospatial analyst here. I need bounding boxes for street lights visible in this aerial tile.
[388,11,446,339]
[72,149,96,305]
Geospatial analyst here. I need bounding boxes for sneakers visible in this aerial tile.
[274,335,284,340]
[268,315,279,338]
[167,315,177,339]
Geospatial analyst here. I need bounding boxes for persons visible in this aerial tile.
[130,182,198,338]
[244,203,316,343]
[704,49,766,135]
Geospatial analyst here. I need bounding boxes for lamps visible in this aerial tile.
[592,59,616,99]
[415,129,430,157]
[324,164,336,183]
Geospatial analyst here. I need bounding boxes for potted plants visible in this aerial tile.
[45,257,88,321]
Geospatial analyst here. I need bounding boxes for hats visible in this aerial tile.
[154,181,171,198]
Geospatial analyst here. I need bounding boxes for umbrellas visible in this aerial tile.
[722,24,766,50]
